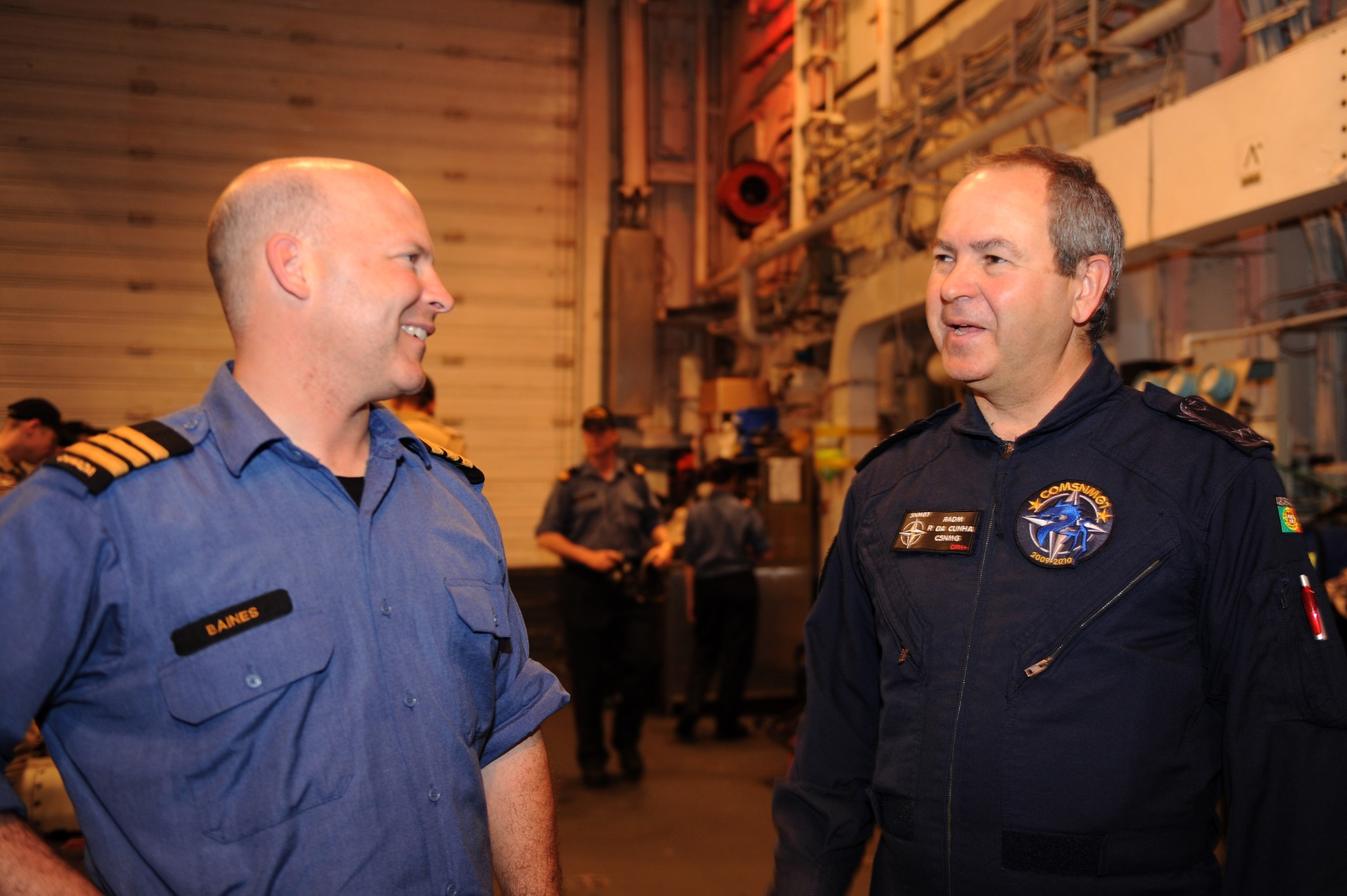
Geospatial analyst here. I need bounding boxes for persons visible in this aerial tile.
[535,405,672,788]
[0,157,569,896]
[764,146,1347,895]
[0,397,112,496]
[392,373,466,457]
[674,457,772,743]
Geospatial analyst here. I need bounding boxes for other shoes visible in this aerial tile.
[615,746,645,779]
[585,762,611,787]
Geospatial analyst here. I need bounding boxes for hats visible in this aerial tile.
[582,406,613,433]
[7,397,64,433]
